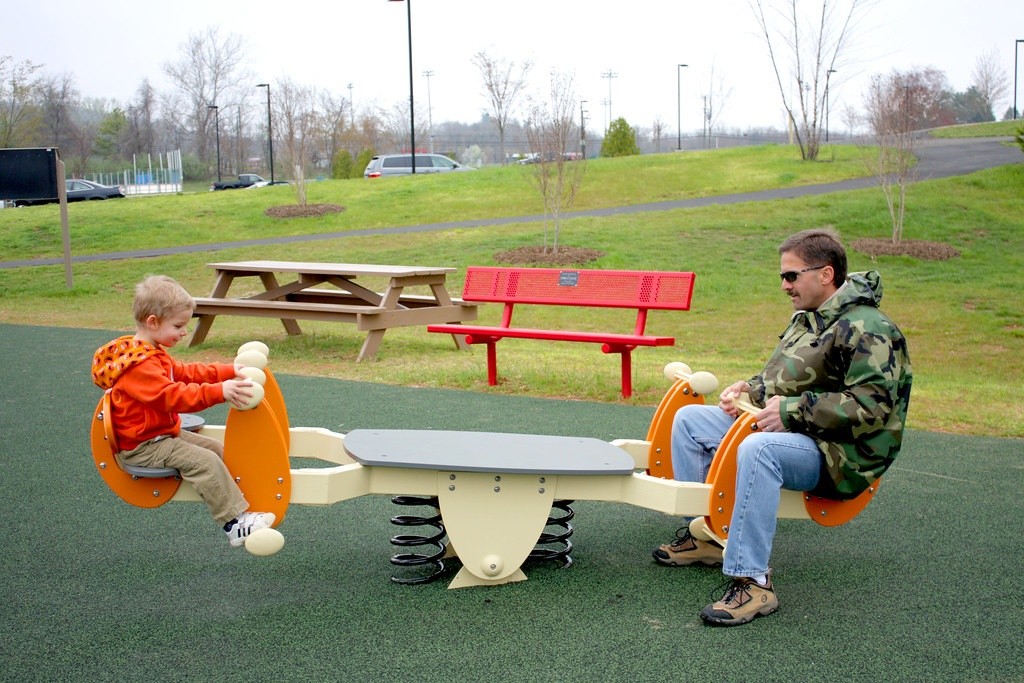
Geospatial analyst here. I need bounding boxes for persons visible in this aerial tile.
[651,225,915,628]
[90,274,276,548]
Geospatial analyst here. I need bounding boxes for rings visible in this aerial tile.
[766,427,770,431]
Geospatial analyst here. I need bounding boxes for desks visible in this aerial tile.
[187,260,472,365]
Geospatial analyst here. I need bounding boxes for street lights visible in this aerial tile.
[390,0,417,175]
[207,105,221,181]
[1012,40,1024,119]
[255,83,276,185]
[580,99,587,159]
[422,69,434,155]
[825,69,838,142]
[676,62,690,151]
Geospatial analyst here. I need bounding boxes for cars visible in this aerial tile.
[517,151,575,168]
[209,173,291,190]
[13,179,126,207]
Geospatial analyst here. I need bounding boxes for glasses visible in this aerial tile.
[779,265,838,283]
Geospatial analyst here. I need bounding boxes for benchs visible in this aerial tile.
[428,265,695,399]
[188,288,485,332]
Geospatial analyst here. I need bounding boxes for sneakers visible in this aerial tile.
[652,522,724,564]
[700,568,779,626]
[223,511,276,548]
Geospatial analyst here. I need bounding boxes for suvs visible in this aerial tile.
[362,153,478,178]
